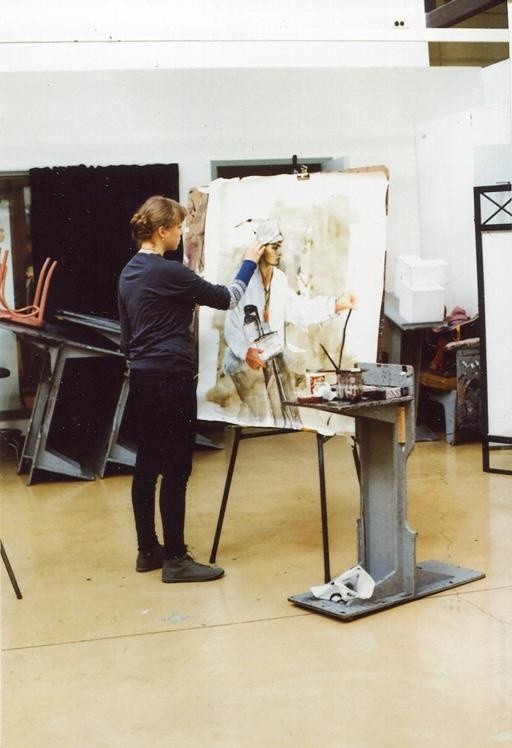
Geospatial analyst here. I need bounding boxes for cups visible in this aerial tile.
[336,368,363,400]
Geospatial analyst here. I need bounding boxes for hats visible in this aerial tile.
[235,218,283,246]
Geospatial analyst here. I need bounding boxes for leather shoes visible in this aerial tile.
[136,544,188,573]
[161,553,224,582]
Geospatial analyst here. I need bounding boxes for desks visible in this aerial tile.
[279,360,484,622]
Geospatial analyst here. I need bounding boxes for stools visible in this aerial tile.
[0,249,58,328]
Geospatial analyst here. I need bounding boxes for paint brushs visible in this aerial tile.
[319,344,339,377]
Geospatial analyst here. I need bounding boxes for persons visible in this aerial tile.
[220,220,356,426]
[117,196,265,584]
